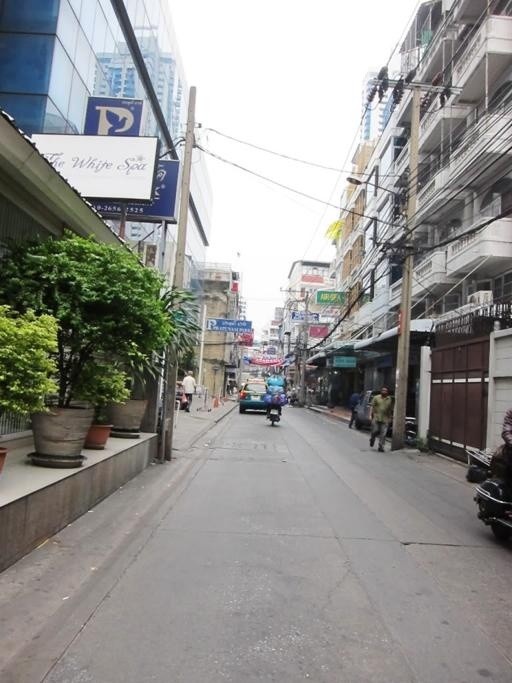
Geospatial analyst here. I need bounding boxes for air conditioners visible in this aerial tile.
[467,290,493,305]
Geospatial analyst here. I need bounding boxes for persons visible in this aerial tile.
[285,385,301,407]
[181,370,198,412]
[349,391,365,428]
[369,385,392,452]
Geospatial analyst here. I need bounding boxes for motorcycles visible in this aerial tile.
[268,395,280,425]
[473,477,512,539]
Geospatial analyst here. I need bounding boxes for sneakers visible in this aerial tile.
[186,408,190,413]
[369,439,386,453]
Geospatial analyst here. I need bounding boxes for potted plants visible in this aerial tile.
[0,228,200,475]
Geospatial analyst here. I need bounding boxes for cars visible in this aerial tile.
[239,379,270,413]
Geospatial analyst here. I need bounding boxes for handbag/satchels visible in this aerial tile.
[181,393,188,404]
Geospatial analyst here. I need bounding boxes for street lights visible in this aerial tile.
[347,176,396,197]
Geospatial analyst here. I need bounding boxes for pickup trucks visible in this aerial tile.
[354,390,394,429]
